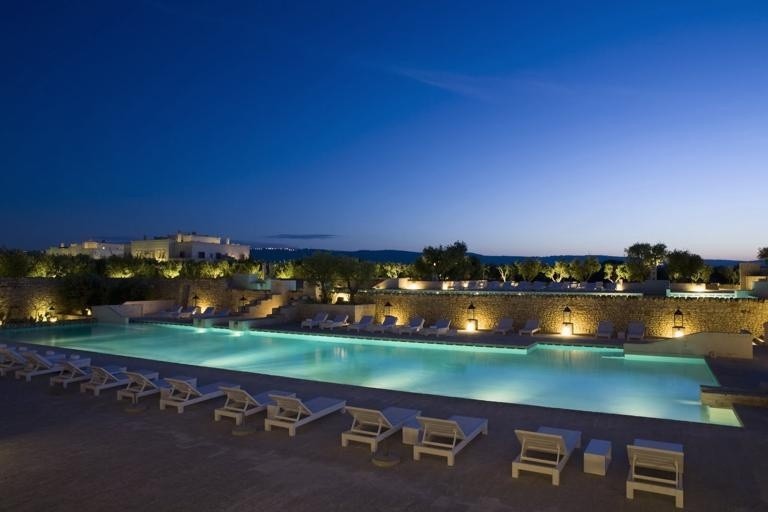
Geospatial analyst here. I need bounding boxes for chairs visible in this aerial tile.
[0,343,159,397]
[340,406,422,453]
[413,415,488,466]
[494,317,517,335]
[595,320,613,340]
[627,321,644,341]
[626,437,684,508]
[159,378,240,414]
[264,395,347,438]
[214,386,297,426]
[151,304,232,322]
[519,319,541,336]
[116,371,197,404]
[511,427,582,486]
[300,311,452,339]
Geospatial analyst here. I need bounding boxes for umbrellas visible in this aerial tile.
[192,296,199,307]
[240,297,247,306]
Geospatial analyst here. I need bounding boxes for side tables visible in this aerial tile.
[402,419,423,445]
[267,401,281,419]
[160,386,173,398]
[583,438,612,477]
[617,331,626,339]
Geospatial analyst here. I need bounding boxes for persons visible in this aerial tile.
[82,306,93,317]
